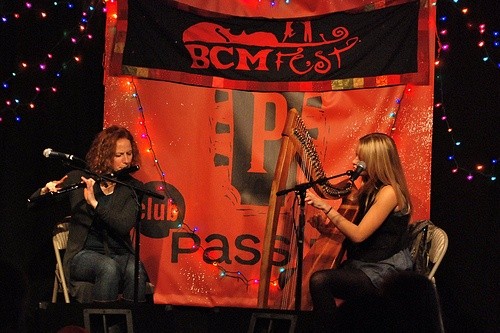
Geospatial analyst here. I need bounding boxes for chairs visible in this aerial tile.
[52,223,153,303]
[411,226,448,333]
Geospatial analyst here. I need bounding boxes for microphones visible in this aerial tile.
[43,148,76,160]
[348,161,366,185]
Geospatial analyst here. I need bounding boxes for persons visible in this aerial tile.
[278,133,412,333]
[27,125,154,333]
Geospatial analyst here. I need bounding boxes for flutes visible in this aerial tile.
[26,165,140,202]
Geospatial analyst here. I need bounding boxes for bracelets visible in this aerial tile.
[325,206,332,214]
